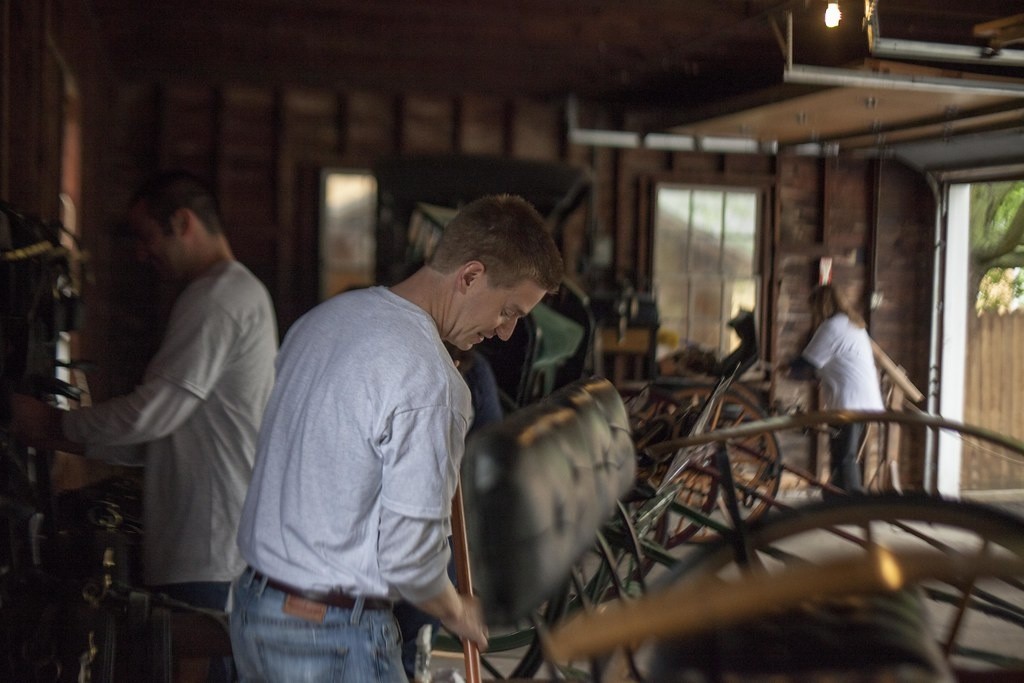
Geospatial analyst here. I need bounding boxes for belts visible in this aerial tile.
[246,565,392,611]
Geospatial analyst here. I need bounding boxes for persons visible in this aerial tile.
[791,282,886,502]
[0,170,279,682]
[228,194,566,683]
